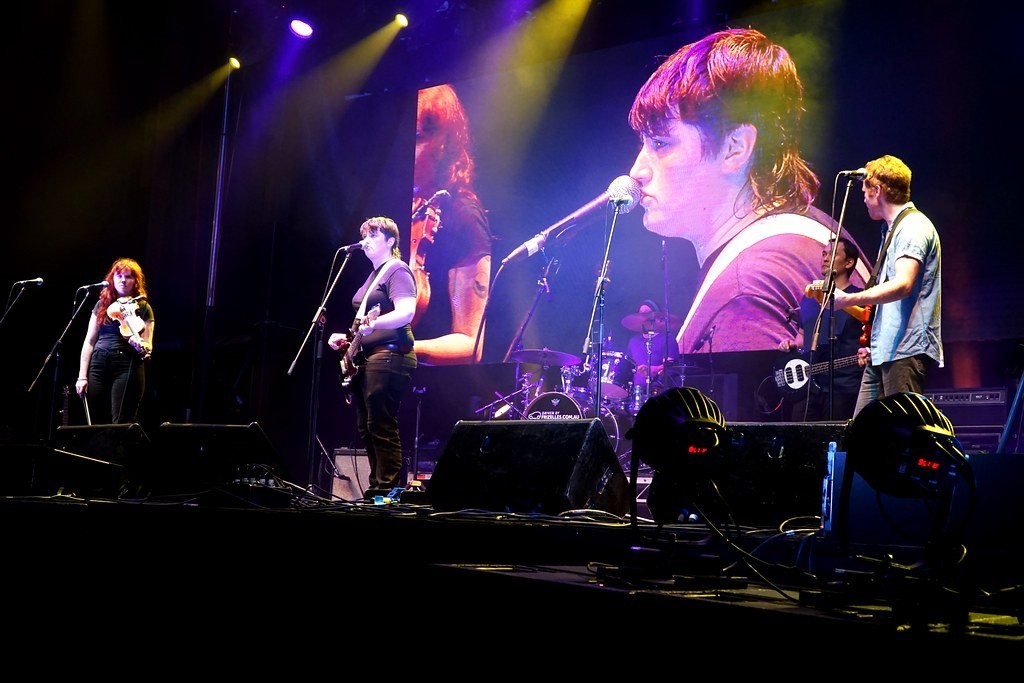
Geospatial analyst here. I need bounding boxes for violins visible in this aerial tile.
[106,295,152,362]
[408,189,444,332]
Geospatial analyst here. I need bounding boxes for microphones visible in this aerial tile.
[83,280,110,289]
[542,276,553,303]
[609,194,635,207]
[344,240,368,251]
[694,326,716,351]
[16,277,44,286]
[839,167,868,181]
[502,174,642,262]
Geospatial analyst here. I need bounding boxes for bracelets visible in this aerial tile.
[77,378,87,380]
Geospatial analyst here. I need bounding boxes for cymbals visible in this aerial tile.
[621,311,683,334]
[509,349,582,366]
[671,365,700,373]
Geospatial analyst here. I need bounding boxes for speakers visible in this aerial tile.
[422,419,629,520]
[646,421,830,532]
[328,446,409,505]
[56,424,152,498]
[152,423,278,499]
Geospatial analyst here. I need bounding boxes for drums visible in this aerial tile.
[587,350,637,401]
[520,391,620,479]
[623,384,652,417]
[553,384,588,400]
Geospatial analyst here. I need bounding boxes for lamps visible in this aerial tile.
[836,391,968,499]
[392,0,451,34]
[624,386,734,512]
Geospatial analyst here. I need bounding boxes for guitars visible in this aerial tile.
[335,302,381,406]
[771,344,859,402]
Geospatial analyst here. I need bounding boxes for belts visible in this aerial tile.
[371,343,414,353]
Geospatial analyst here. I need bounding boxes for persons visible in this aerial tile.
[778,236,867,420]
[413,84,491,365]
[329,216,417,504]
[826,155,944,421]
[76,260,154,425]
[629,29,873,354]
[625,299,680,385]
